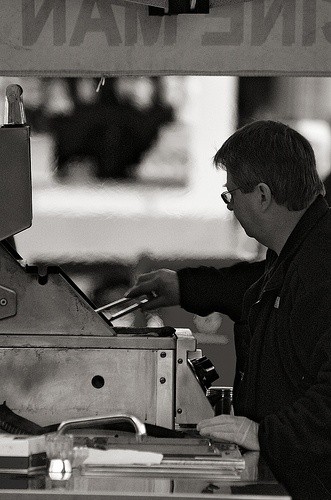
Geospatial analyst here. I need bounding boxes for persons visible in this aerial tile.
[124,119,331,500]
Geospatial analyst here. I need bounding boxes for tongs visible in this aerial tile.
[94,291,161,321]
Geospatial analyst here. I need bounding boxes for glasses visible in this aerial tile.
[221,182,254,203]
